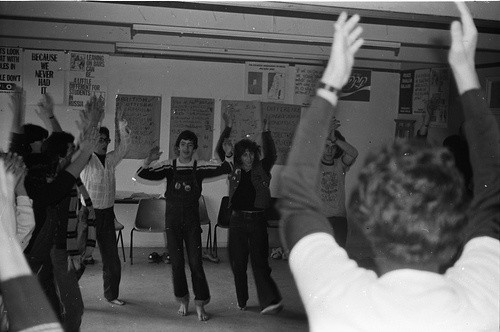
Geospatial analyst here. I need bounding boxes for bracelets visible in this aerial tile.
[332,137,337,143]
[423,123,428,127]
[49,115,54,119]
[316,81,342,97]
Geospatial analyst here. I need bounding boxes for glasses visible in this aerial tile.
[98,137,112,144]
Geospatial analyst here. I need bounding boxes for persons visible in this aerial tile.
[215,105,284,314]
[416,102,433,138]
[136,130,226,321]
[432,133,473,196]
[319,116,358,249]
[0,87,132,332]
[280,1,500,332]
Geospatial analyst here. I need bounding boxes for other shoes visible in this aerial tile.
[148,251,170,264]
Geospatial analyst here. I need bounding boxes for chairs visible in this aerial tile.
[266,197,283,227]
[213,194,235,258]
[115,210,126,262]
[198,195,212,260]
[129,199,170,264]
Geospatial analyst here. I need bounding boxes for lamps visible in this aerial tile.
[115,41,402,63]
[131,24,401,50]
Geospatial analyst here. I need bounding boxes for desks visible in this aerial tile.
[115,192,167,204]
